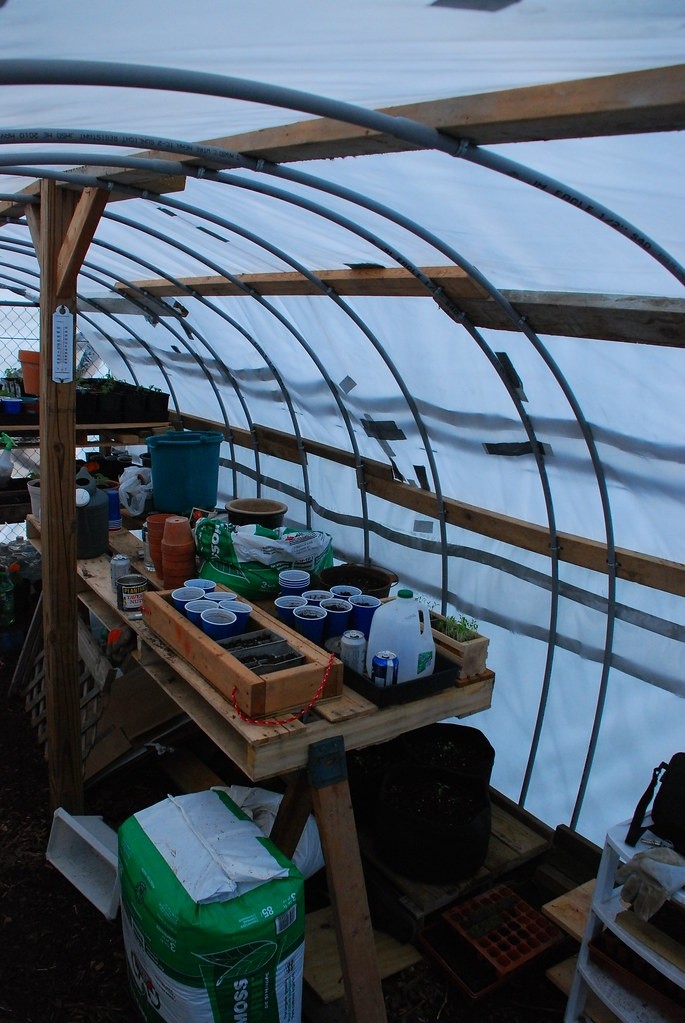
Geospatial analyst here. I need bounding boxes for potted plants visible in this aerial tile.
[94,474,120,491]
[379,595,490,681]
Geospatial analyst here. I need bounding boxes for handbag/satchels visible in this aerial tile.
[118,464,154,516]
[625,753,685,856]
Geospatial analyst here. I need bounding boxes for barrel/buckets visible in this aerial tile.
[145,428,224,513]
[319,560,399,599]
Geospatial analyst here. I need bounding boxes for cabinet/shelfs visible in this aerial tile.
[563,809,685,1023]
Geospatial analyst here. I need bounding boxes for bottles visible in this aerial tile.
[365,589,435,688]
[143,520,157,572]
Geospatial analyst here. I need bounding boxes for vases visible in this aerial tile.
[224,498,288,530]
[27,479,41,523]
[146,513,254,645]
[271,562,398,646]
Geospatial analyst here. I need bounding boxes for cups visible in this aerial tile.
[274,595,308,629]
[183,578,216,594]
[321,598,353,638]
[204,591,238,603]
[184,600,219,629]
[172,587,205,615]
[219,601,252,635]
[293,604,327,643]
[107,490,122,531]
[201,608,237,642]
[348,595,382,641]
[330,585,363,601]
[302,590,334,607]
[278,570,310,596]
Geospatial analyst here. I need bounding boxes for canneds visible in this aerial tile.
[371,650,399,688]
[339,630,366,677]
[110,554,130,592]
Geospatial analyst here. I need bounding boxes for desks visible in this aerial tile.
[0,508,498,1023]
